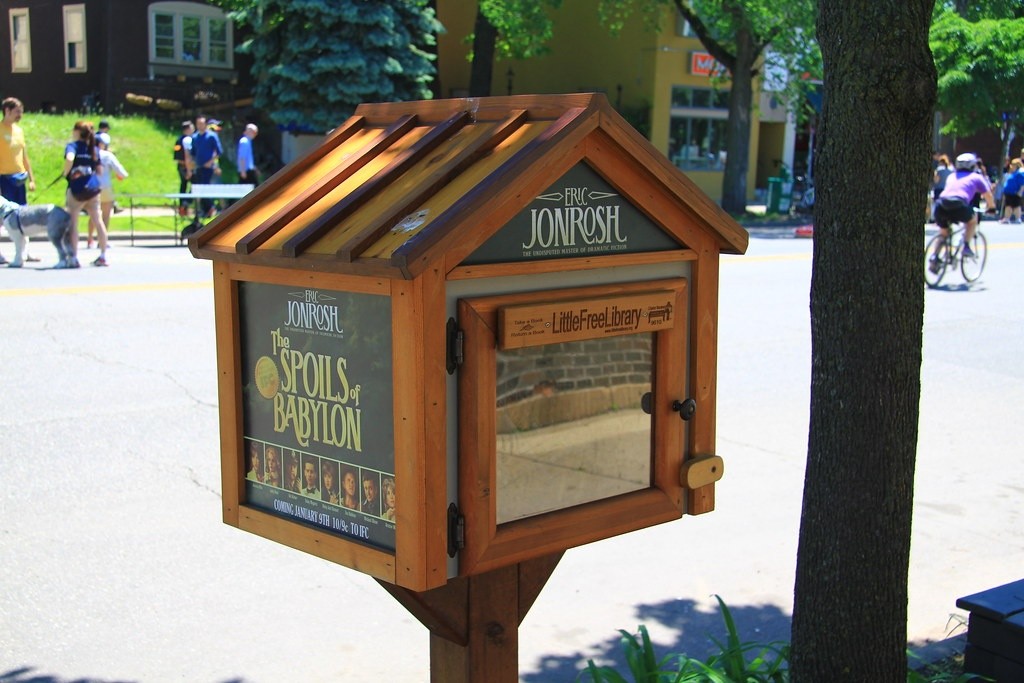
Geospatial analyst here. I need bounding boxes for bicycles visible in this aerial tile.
[921,203,992,288]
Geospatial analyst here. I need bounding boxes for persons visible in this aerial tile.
[283,449,302,494]
[361,473,380,517]
[0,97,41,264]
[928,151,997,275]
[265,447,283,488]
[172,113,225,218]
[321,461,340,505]
[246,441,265,483]
[302,457,320,500]
[341,467,360,512]
[81,121,124,214]
[86,133,128,250]
[382,477,396,523]
[225,123,261,208]
[681,135,700,162]
[934,148,1024,223]
[62,121,109,268]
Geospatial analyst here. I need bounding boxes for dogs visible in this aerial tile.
[0,196,82,270]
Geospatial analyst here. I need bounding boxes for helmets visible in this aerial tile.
[956,152,975,169]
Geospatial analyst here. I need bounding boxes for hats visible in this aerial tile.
[94,132,110,144]
[206,118,223,126]
[206,124,221,133]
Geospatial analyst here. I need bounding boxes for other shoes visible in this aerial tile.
[67,256,80,269]
[929,255,939,275]
[0,254,8,264]
[998,217,1021,224]
[87,239,111,250]
[91,256,106,266]
[962,242,974,257]
[176,203,226,219]
[26,254,40,262]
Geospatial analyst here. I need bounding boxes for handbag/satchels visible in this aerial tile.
[67,165,102,203]
[4,170,29,188]
